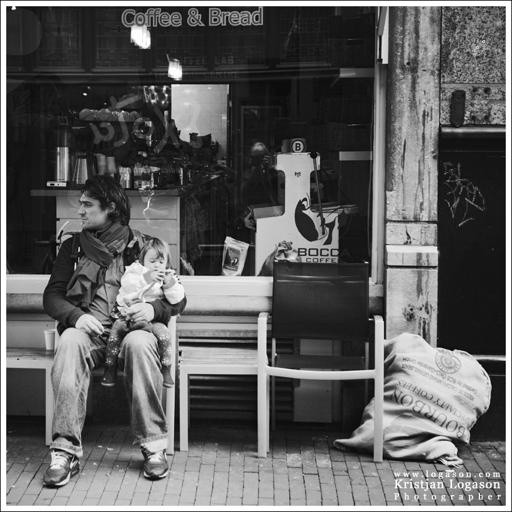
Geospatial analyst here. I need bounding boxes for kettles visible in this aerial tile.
[72,151,96,186]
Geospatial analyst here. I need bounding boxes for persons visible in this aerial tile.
[42,175,187,489]
[99,236,185,388]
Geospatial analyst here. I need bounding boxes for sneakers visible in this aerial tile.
[43,449,79,486]
[161,364,174,388]
[101,363,117,387]
[141,446,170,479]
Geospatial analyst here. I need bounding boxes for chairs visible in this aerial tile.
[257,259,385,462]
[190,182,232,273]
[44,315,189,457]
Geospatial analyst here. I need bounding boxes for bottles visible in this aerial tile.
[133,162,142,188]
[141,165,151,189]
[118,161,131,189]
[57,147,70,182]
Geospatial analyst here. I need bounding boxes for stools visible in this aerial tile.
[34,226,56,272]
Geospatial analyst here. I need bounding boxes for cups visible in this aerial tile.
[97,155,106,174]
[43,329,56,349]
[106,156,116,174]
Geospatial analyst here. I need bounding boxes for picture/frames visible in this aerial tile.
[237,100,284,212]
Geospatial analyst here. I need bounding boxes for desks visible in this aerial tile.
[30,174,220,275]
